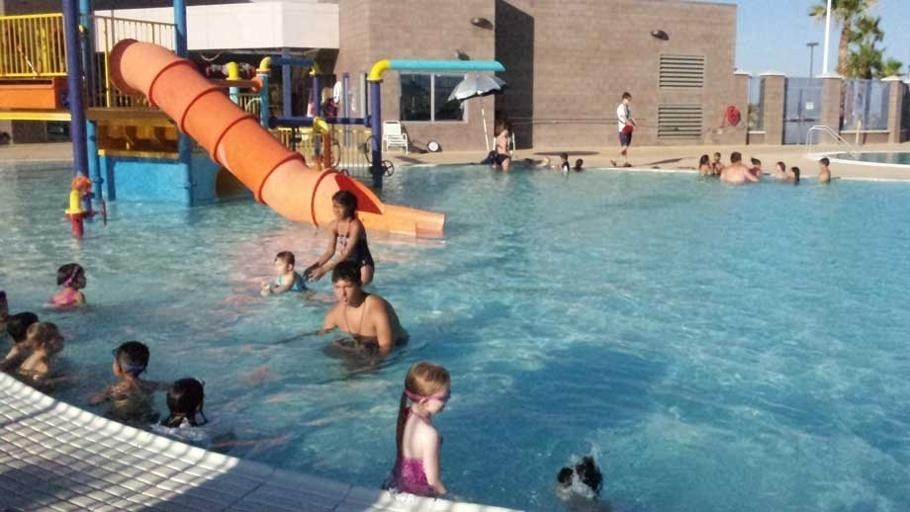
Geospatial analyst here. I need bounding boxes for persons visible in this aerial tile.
[266,191,410,364]
[555,467,574,500]
[306,95,316,117]
[323,98,336,117]
[1,264,208,429]
[480,119,583,174]
[819,158,830,183]
[383,363,450,496]
[610,93,636,168]
[573,456,603,501]
[698,151,799,184]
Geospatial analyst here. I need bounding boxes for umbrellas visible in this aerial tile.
[447,73,508,151]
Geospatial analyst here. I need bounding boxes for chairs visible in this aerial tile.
[382,120,408,157]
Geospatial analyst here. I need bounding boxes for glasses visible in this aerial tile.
[404,387,451,401]
